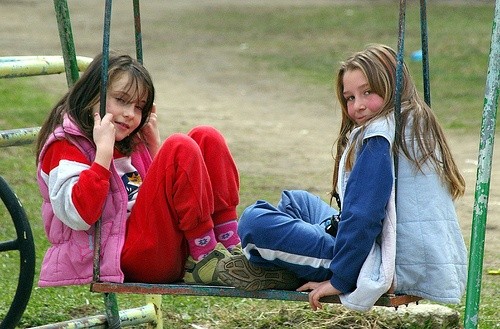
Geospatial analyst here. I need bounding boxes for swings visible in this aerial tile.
[90,0,434,309]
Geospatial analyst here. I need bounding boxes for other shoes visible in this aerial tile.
[216,254,300,291]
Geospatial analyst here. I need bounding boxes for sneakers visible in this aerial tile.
[228,243,243,256]
[183,242,232,284]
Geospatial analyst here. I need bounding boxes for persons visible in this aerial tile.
[216,43,469,312]
[35,48,244,288]
